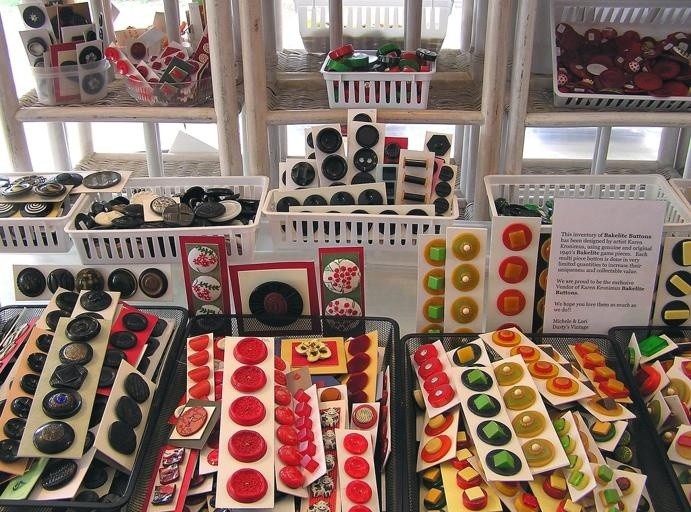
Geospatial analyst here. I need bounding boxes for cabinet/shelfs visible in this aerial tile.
[500,0,691,179]
[237,0,510,220]
[0,0,244,177]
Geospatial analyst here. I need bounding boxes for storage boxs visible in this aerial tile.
[262,188,460,250]
[319,48,437,110]
[63,175,270,265]
[30,56,110,105]
[608,325,691,512]
[0,304,191,512]
[294,0,455,54]
[120,313,401,512]
[483,173,691,238]
[124,76,212,109]
[668,178,691,212]
[401,332,691,512]
[0,170,99,254]
[548,0,691,112]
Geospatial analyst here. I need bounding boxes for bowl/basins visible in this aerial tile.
[30,55,111,105]
[122,72,212,108]
